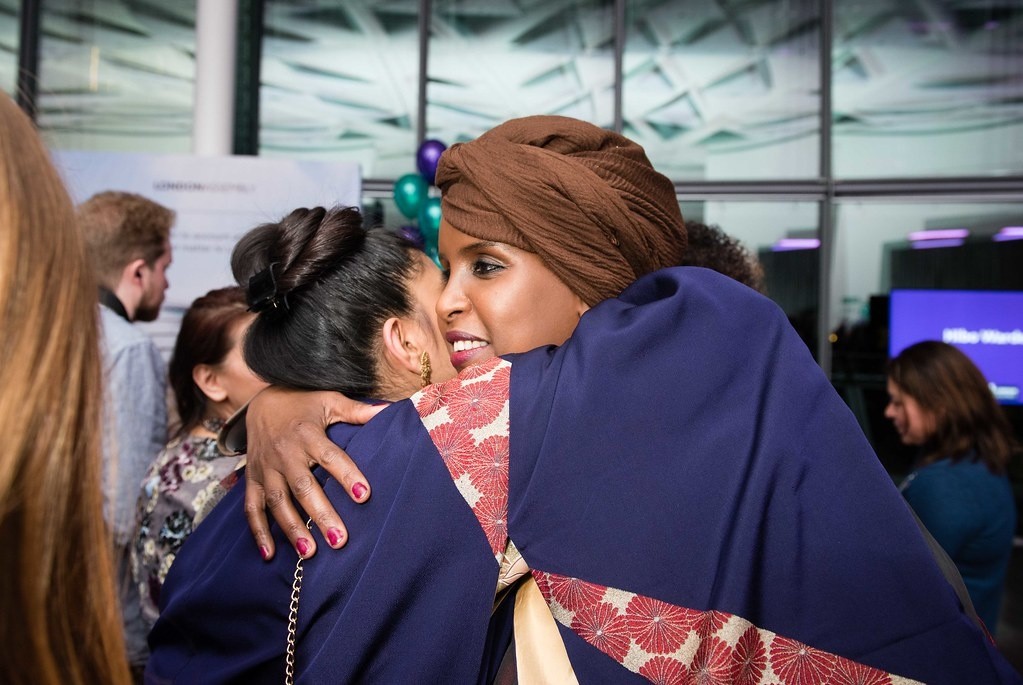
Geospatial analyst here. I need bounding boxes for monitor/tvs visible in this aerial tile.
[890,288,1023,406]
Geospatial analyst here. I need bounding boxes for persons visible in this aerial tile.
[144,203,783,684]
[63,186,177,596]
[883,339,1015,656]
[122,286,271,647]
[218,115,1022,684]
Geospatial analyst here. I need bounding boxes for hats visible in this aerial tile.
[432,114,689,311]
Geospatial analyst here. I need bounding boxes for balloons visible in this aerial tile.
[392,139,451,276]
